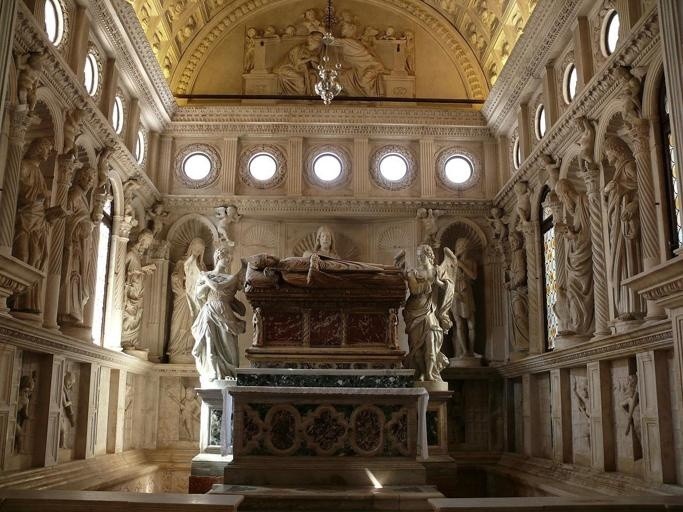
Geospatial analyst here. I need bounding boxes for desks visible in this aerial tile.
[0,488,245,512]
[427,495,683,512]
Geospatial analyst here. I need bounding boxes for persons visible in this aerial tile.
[402,245,457,382]
[214,205,243,242]
[183,246,248,381]
[10,48,207,367]
[14,375,35,454]
[444,65,648,361]
[301,225,341,259]
[248,252,407,288]
[179,386,201,441]
[242,4,415,95]
[619,373,641,445]
[59,372,76,448]
[416,208,446,245]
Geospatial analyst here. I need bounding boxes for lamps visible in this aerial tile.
[314,1,343,105]
